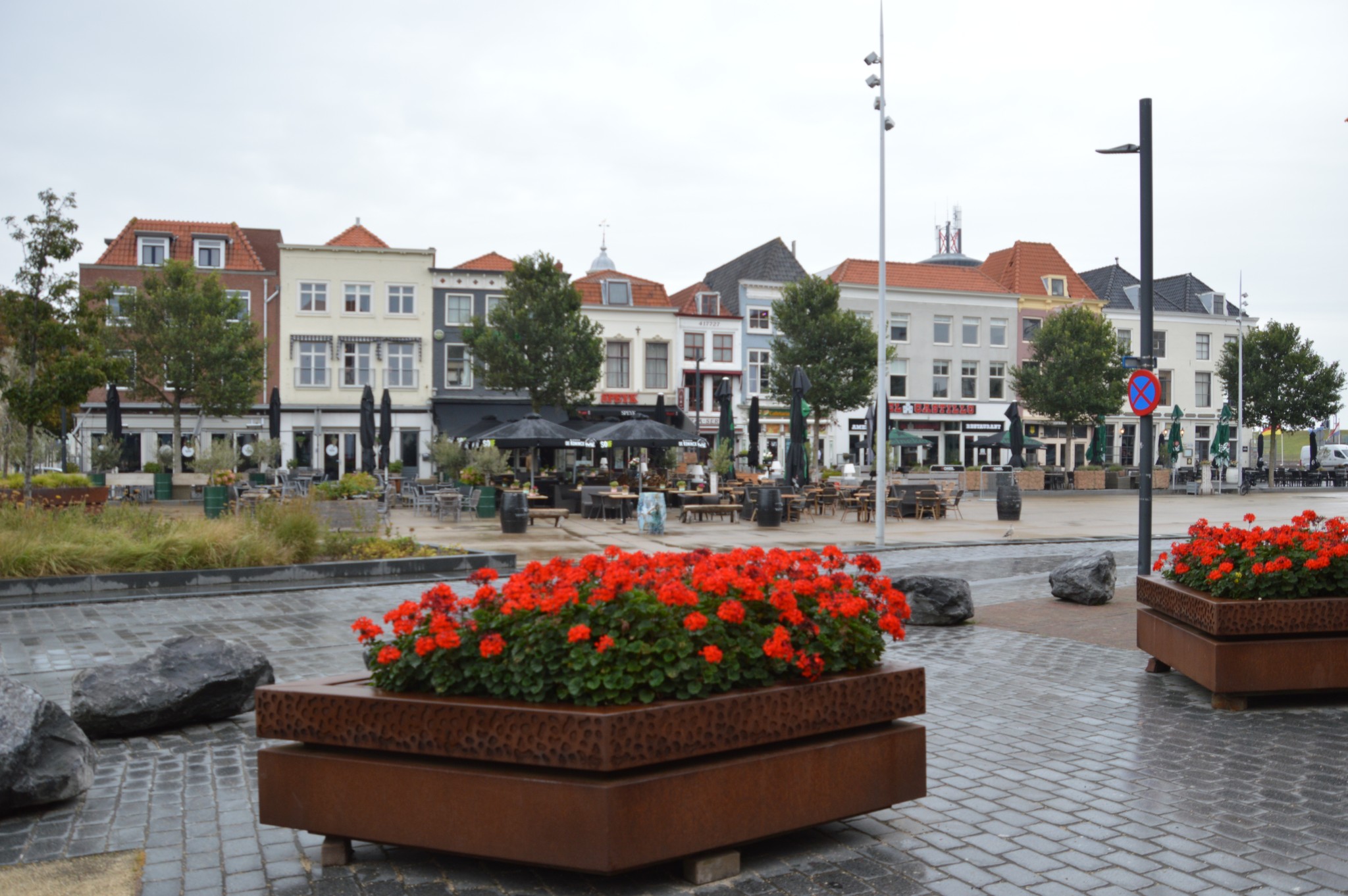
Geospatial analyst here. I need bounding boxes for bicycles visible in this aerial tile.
[1239,470,1256,496]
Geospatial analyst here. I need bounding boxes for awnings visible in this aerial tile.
[569,406,696,434]
[434,399,569,436]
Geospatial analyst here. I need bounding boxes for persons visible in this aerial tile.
[647,505,663,534]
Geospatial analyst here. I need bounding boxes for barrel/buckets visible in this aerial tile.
[88,473,105,485]
[997,486,1021,520]
[756,489,782,527]
[204,485,228,519]
[249,472,265,485]
[475,486,496,518]
[500,493,529,533]
[637,492,667,535]
[154,473,172,500]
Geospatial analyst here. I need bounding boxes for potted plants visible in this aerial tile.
[501,477,508,488]
[609,480,619,493]
[521,481,532,493]
[1108,462,1126,477]
[1151,466,1171,491]
[1015,464,1046,492]
[510,479,520,490]
[959,465,989,492]
[675,479,688,491]
[285,457,300,478]
[387,458,404,494]
[1072,464,1106,490]
[659,480,666,489]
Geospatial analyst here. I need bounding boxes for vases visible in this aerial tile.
[621,490,629,495]
[531,490,539,496]
[1133,571,1348,713]
[696,486,704,493]
[251,662,936,886]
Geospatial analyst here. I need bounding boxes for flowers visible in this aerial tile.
[211,466,236,485]
[1151,503,1348,598]
[343,541,921,680]
[458,465,484,486]
[532,486,538,490]
[622,483,630,490]
[697,482,705,489]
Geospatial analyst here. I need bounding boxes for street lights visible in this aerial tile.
[1094,95,1155,576]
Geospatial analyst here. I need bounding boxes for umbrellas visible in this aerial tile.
[1085,415,1106,465]
[587,413,709,494]
[456,413,624,526]
[747,396,759,472]
[785,365,812,492]
[1004,400,1026,467]
[1212,457,1219,481]
[855,427,935,472]
[1210,404,1232,494]
[865,390,890,466]
[1155,433,1166,465]
[1168,404,1184,490]
[269,386,283,487]
[1310,432,1321,470]
[714,377,736,482]
[1257,434,1264,469]
[379,388,392,484]
[105,384,122,499]
[361,383,376,476]
[969,431,1047,464]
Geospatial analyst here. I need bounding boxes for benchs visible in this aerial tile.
[526,506,571,528]
[579,483,633,519]
[890,482,949,519]
[679,501,744,526]
[0,484,112,517]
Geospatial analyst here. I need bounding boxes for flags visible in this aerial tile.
[1261,422,1283,436]
[1326,421,1340,443]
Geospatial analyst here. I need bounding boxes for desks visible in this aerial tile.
[606,493,638,525]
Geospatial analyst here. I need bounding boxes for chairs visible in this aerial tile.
[1066,471,1074,489]
[233,466,483,524]
[1173,461,1348,489]
[641,471,968,526]
[587,491,606,524]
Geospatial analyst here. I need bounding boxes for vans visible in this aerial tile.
[1299,443,1348,480]
